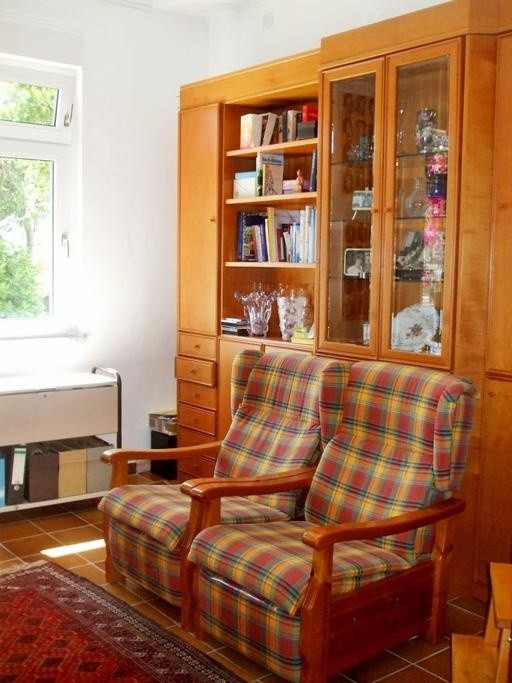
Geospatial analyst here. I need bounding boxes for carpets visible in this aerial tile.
[0,559,250,683]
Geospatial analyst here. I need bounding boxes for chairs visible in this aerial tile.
[186,359,481,683]
[97,349,356,632]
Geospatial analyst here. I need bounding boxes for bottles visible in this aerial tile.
[405,175,432,218]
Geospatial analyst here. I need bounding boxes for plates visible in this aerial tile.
[393,302,439,351]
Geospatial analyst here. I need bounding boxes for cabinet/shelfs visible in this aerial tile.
[0,365,122,515]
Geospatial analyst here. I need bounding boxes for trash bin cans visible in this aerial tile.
[148,409,178,480]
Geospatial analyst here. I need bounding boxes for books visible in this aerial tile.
[232,104,317,262]
[221,317,247,336]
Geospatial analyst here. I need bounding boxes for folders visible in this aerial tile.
[0,444,28,508]
